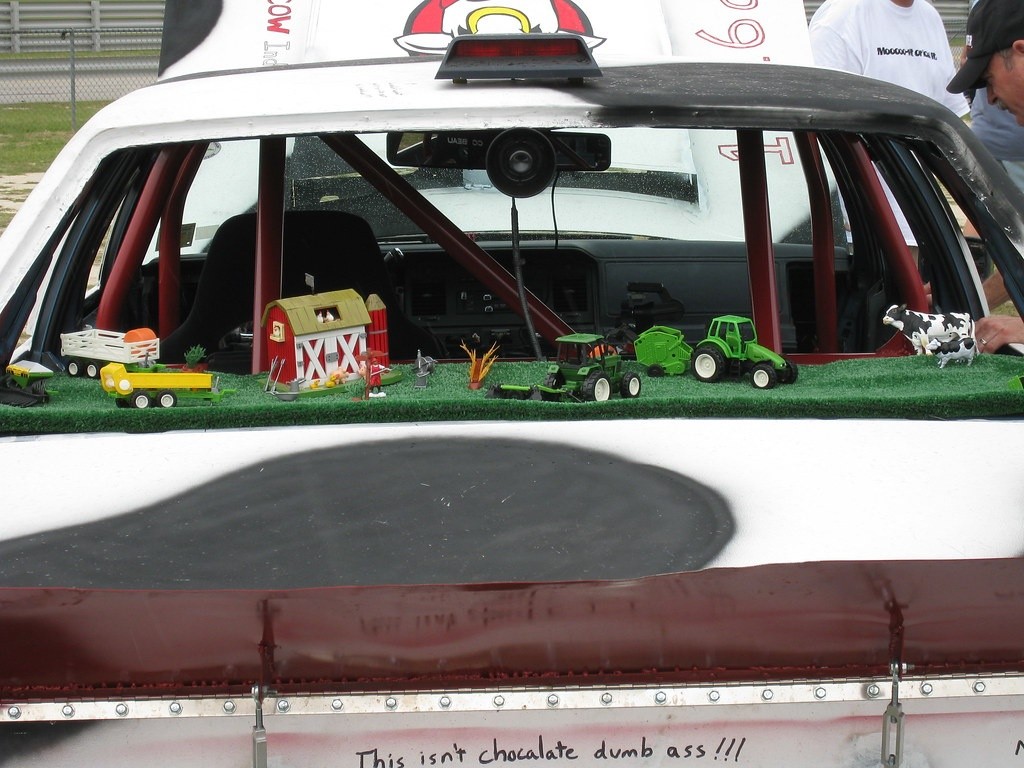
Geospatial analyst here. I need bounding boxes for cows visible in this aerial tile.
[883,303,981,358]
[925,337,976,369]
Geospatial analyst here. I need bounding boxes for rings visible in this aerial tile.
[978,337,987,345]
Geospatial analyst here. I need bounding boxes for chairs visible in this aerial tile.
[160,208,446,374]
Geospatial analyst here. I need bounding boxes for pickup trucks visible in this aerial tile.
[0,39,1024,768]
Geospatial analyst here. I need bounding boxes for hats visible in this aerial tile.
[946,0,1024,94]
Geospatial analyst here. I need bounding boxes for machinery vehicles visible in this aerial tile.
[486,333,642,402]
[634,314,799,390]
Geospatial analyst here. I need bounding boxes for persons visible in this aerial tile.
[947,0,1024,354]
[808,0,971,247]
